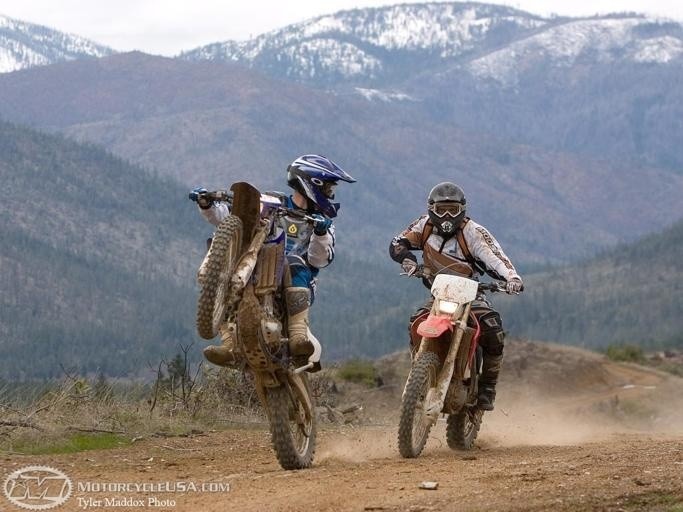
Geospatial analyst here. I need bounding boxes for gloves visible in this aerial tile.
[311,213,332,236]
[506,277,524,295]
[401,253,418,277]
[189,186,212,210]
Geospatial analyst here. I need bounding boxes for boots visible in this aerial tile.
[202,322,240,371]
[476,310,506,411]
[285,286,314,357]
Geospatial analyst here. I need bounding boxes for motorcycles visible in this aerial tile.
[187,182,326,469]
[398,263,523,457]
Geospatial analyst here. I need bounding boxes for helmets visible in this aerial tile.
[286,154,357,218]
[427,181,467,235]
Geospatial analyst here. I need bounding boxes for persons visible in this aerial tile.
[188,153,357,367]
[390,181,523,411]
